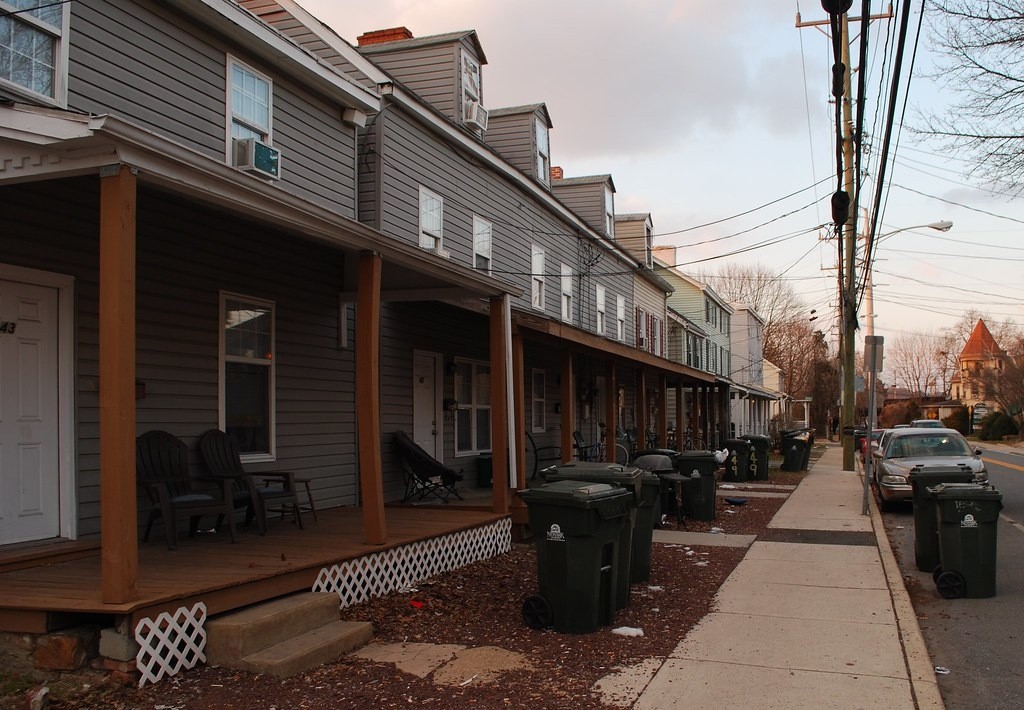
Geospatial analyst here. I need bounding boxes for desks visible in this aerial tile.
[263,478,318,523]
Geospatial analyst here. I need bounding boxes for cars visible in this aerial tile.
[854,419,990,511]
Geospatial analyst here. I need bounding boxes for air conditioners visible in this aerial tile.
[238,140,282,182]
[639,336,648,349]
[468,102,488,132]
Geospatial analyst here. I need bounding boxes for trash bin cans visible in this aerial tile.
[778,428,816,471]
[631,470,659,581]
[515,479,634,633]
[721,439,749,482]
[855,431,868,452]
[676,450,717,520]
[538,462,643,577]
[737,435,771,480]
[925,482,1002,601]
[634,455,673,515]
[633,448,681,467]
[909,464,976,569]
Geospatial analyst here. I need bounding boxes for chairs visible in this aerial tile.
[525,431,563,480]
[135,430,241,549]
[198,429,304,537]
[394,431,464,505]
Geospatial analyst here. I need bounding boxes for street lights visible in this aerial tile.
[841,220,954,471]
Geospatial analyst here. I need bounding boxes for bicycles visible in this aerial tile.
[598,425,708,468]
